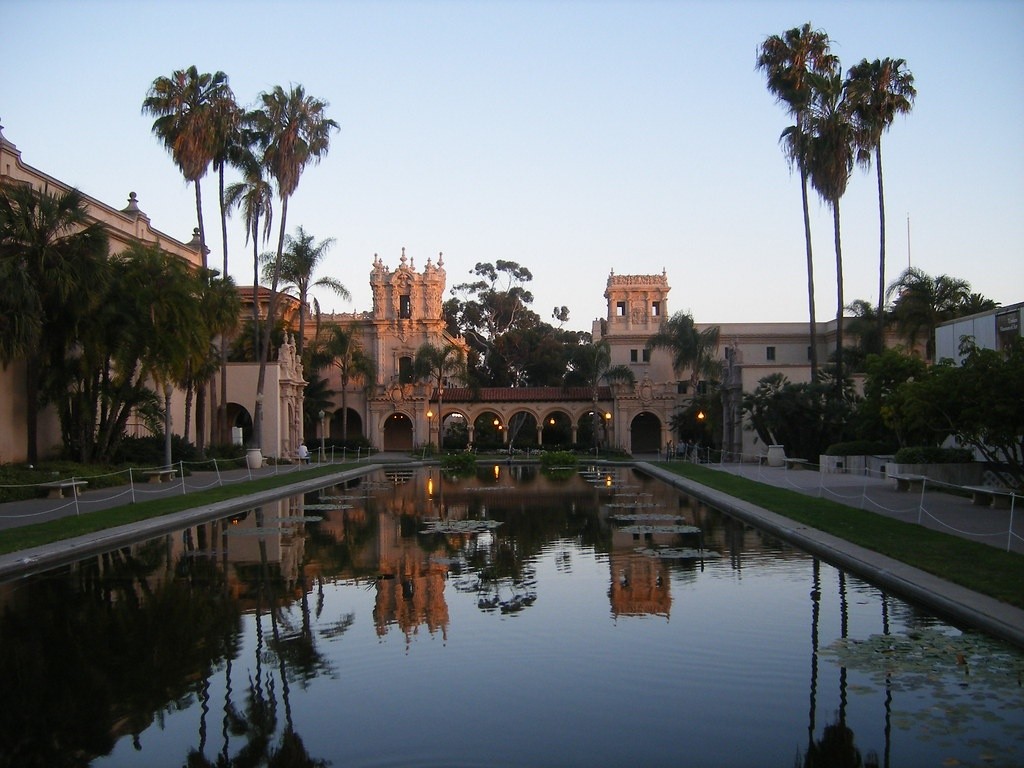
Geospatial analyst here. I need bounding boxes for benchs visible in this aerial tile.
[47,481,88,499]
[291,457,309,464]
[261,458,268,467]
[756,455,768,466]
[961,485,1022,509]
[143,470,178,483]
[782,458,808,470]
[888,474,929,492]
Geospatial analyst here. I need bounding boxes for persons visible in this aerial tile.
[667,439,694,460]
[299,443,311,464]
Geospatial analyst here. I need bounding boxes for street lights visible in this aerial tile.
[697,411,706,460]
[550,418,556,445]
[605,412,612,450]
[426,410,433,445]
[255,391,264,448]
[493,418,499,449]
[318,410,328,464]
[162,382,174,471]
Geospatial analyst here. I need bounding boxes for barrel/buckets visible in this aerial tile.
[767,445,786,467]
[245,449,262,469]
